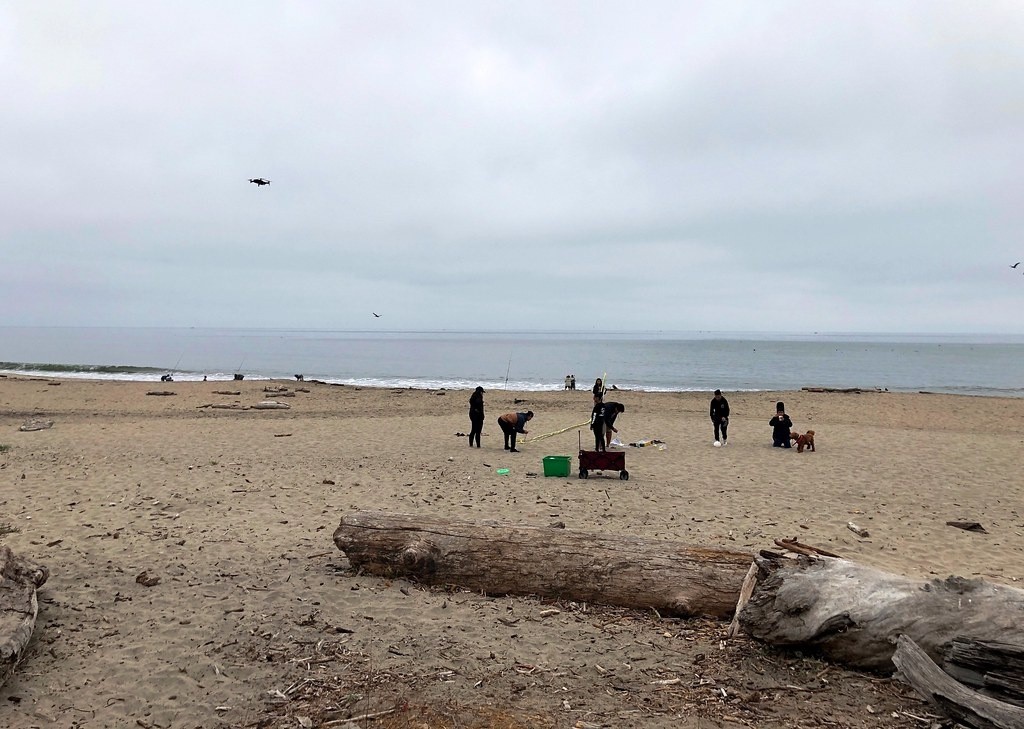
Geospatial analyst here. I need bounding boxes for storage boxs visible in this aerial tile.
[542,455,572,477]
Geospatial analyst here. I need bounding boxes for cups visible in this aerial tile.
[779,416,784,421]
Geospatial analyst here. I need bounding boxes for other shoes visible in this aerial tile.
[505,446,511,450]
[723,439,727,445]
[510,449,520,452]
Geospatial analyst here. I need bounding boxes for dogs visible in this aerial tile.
[789,430,815,453]
[295,374,304,382]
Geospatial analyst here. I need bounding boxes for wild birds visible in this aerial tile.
[372,313,382,317]
[1009,262,1020,268]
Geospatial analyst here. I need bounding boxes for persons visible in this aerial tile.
[590,402,624,451]
[709,390,730,446]
[204,376,207,381]
[295,374,303,381]
[769,410,793,448]
[565,375,575,390]
[469,386,486,448]
[498,410,534,452]
[161,374,173,381]
[593,378,607,403]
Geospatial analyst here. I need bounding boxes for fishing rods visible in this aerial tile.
[503,351,512,391]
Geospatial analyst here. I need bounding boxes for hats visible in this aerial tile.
[777,401,784,411]
[476,386,485,393]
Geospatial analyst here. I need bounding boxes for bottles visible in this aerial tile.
[652,440,667,450]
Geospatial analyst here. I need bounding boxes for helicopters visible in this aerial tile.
[249,178,271,186]
